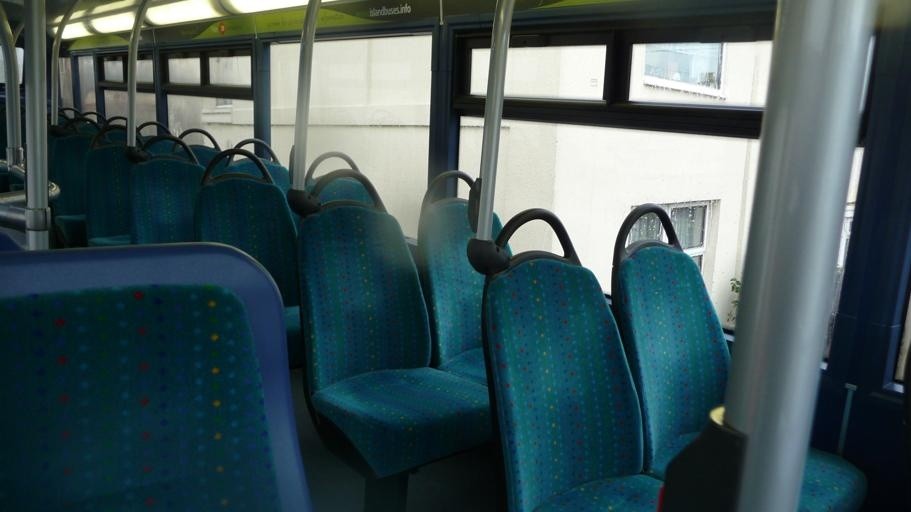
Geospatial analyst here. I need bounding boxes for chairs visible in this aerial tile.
[0,78,868,512]
